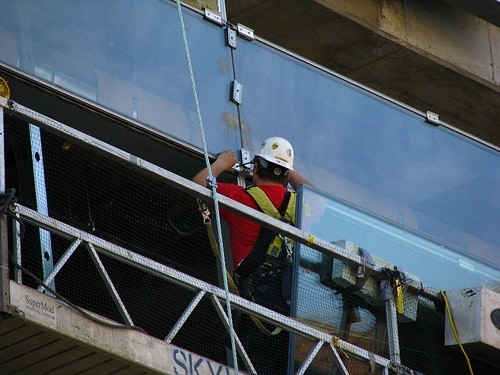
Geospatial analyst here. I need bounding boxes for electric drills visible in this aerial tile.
[235,158,288,187]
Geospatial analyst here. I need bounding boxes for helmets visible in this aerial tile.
[254,137,294,171]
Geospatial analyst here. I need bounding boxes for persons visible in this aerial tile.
[192,137,325,375]
